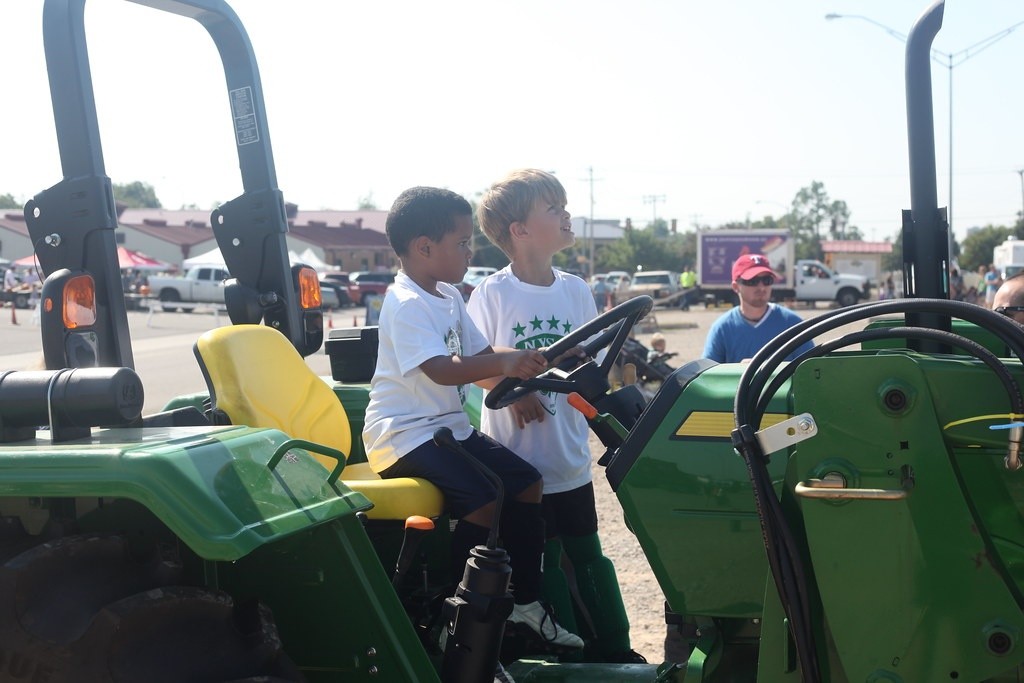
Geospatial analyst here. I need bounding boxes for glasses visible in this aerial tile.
[994,306,1024,315]
[738,276,774,287]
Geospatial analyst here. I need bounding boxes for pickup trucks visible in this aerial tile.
[147,264,240,314]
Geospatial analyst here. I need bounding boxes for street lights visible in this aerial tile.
[825,13,1023,285]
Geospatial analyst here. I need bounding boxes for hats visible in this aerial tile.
[731,254,778,282]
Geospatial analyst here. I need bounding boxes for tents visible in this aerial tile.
[14,244,165,269]
[182,242,340,272]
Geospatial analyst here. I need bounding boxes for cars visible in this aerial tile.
[628,271,680,309]
[452,283,475,303]
[318,272,397,311]
[564,268,632,303]
[462,266,497,290]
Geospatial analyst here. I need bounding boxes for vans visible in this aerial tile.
[991,235,1024,282]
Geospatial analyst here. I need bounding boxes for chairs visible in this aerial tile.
[193,323,439,587]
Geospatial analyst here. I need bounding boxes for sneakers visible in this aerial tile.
[440,623,515,683]
[508,600,585,652]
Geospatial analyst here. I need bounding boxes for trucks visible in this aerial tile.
[694,227,871,310]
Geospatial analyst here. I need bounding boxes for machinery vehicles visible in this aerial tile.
[0,0,1024,683]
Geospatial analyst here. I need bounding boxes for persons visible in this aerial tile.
[118,262,184,292]
[978,262,1024,325]
[5,267,39,290]
[877,273,894,300]
[701,254,815,367]
[361,185,585,652]
[616,276,628,302]
[591,277,606,314]
[681,266,697,311]
[462,170,648,663]
[950,268,963,300]
